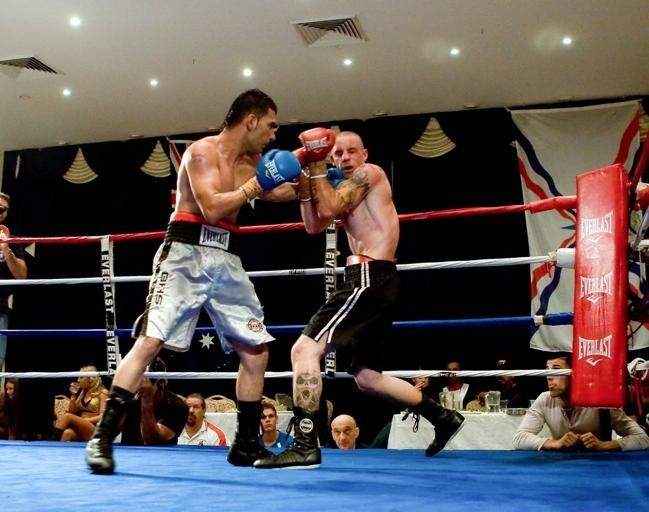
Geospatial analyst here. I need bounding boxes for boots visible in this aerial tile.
[407,393,466,459]
[85,393,134,473]
[252,406,322,470]
[226,397,274,467]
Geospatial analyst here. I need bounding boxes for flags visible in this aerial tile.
[511,101,649,351]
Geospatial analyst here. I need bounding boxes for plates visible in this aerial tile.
[506,409,527,415]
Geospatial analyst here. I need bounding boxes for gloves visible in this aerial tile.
[294,127,345,188]
[255,147,300,193]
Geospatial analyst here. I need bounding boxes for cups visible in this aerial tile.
[530,399,537,405]
[453,401,460,410]
[439,391,455,410]
[275,394,288,406]
[485,391,501,411]
[501,400,508,410]
[72,382,80,393]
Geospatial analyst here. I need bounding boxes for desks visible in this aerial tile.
[387,407,617,452]
[203,410,298,448]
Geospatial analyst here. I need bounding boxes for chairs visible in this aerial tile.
[325,399,334,448]
[260,395,269,400]
[204,394,236,413]
[54,395,71,419]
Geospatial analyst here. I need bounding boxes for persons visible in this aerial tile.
[1,192,28,375]
[252,129,466,470]
[113,358,226,447]
[412,352,648,453]
[259,404,293,449]
[0,366,109,442]
[83,88,342,473]
[330,413,359,448]
